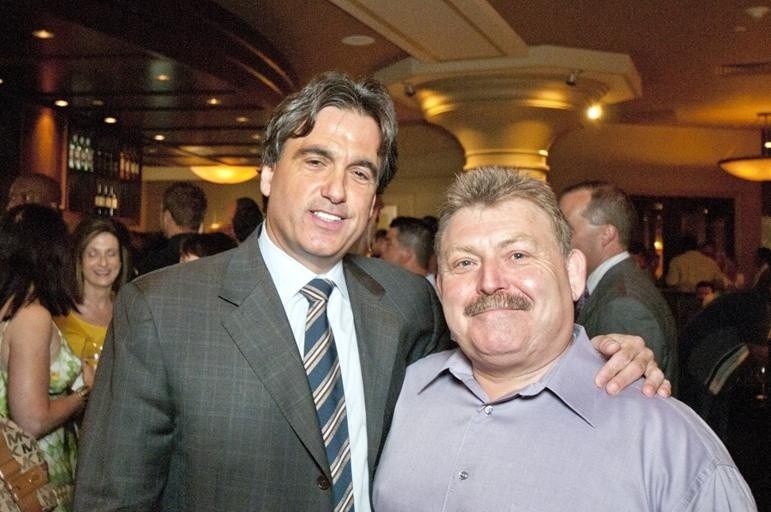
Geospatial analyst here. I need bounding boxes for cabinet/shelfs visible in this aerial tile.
[61,119,142,225]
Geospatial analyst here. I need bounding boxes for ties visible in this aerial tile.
[298,276,355,512]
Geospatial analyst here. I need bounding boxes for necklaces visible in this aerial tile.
[81,296,112,311]
[84,302,110,327]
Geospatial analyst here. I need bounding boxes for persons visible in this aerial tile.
[749,248,771,290]
[553,176,682,402]
[662,238,734,295]
[179,232,237,264]
[366,163,760,511]
[5,174,59,222]
[1,202,96,511]
[347,197,438,285]
[226,196,264,245]
[688,279,716,321]
[46,215,139,373]
[133,183,210,273]
[69,68,675,511]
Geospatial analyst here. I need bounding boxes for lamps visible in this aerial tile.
[404,81,415,96]
[719,112,771,181]
[567,68,582,85]
[190,165,261,184]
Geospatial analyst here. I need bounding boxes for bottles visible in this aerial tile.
[68,128,140,218]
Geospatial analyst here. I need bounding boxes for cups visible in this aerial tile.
[80,336,103,373]
